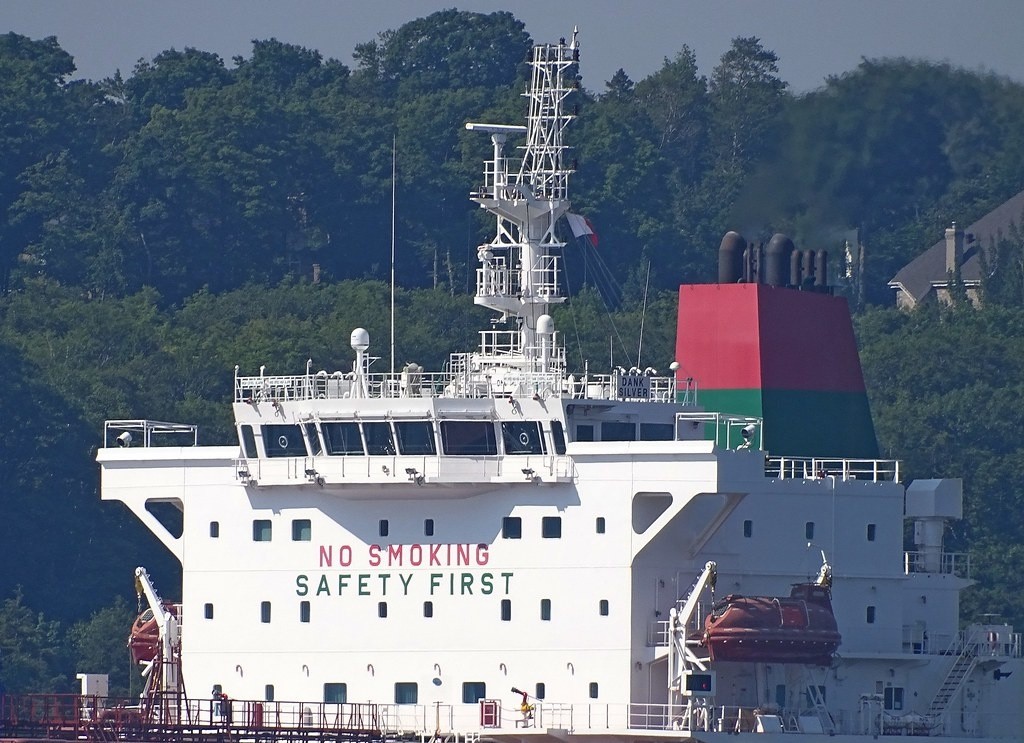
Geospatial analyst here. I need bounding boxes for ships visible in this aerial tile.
[93,20,1024,742]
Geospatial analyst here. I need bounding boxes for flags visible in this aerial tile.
[566,212,599,247]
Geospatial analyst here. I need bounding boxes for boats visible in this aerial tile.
[126,601,179,675]
[701,582,846,666]
